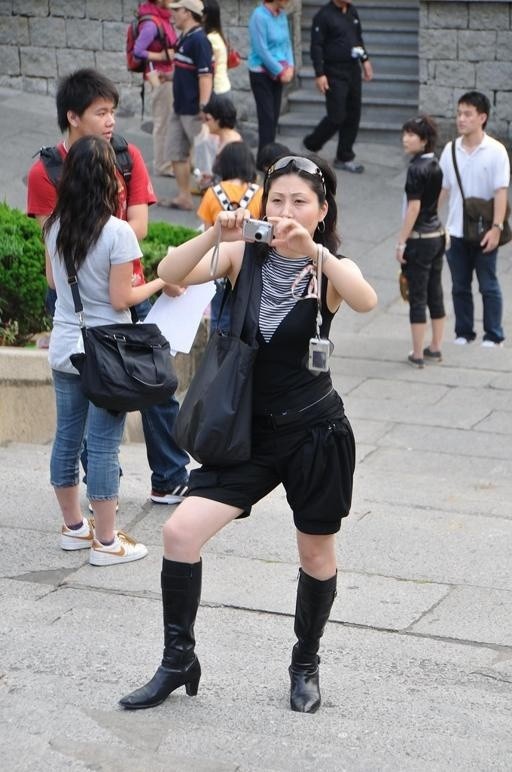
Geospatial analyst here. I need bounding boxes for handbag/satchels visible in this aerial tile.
[461,196,512,248]
[169,238,263,468]
[74,320,181,415]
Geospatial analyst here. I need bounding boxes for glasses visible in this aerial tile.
[263,154,330,199]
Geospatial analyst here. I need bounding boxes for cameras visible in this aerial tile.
[242,218,272,243]
[351,46,364,58]
[308,338,330,372]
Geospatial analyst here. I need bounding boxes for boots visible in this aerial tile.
[117,570,201,711]
[287,569,338,713]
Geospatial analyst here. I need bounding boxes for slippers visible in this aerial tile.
[155,196,195,212]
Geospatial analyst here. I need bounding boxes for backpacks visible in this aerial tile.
[125,9,173,74]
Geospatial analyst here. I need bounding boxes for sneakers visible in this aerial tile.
[57,518,94,552]
[423,346,444,364]
[147,473,193,506]
[478,339,503,347]
[333,157,366,175]
[88,529,150,568]
[450,336,475,346]
[407,349,426,370]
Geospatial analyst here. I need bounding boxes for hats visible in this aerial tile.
[166,0,206,17]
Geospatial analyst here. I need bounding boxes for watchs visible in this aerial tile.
[491,223,504,231]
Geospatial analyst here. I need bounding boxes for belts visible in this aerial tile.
[407,228,445,239]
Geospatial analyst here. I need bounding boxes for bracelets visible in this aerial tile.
[396,243,407,252]
[313,247,329,268]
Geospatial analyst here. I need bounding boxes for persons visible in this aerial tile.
[27,69,190,504]
[197,141,266,339]
[438,92,510,347]
[119,153,377,714]
[395,115,446,369]
[43,135,186,566]
